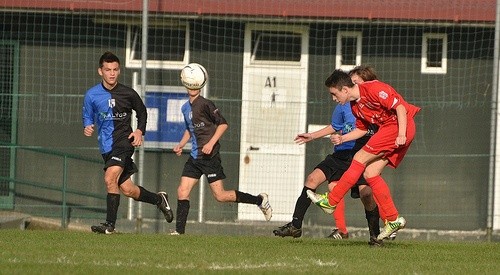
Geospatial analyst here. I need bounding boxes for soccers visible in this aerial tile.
[181,62,208,90]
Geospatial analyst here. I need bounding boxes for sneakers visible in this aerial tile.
[91,222,116,235]
[157,192,173,222]
[368,240,383,246]
[273,223,302,238]
[307,190,336,215]
[258,193,272,221]
[376,217,406,242]
[326,229,348,240]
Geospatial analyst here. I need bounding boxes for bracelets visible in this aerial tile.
[339,136,342,144]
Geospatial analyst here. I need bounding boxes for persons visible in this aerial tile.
[306,70,420,242]
[82,53,173,233]
[273,67,382,246]
[169,89,272,236]
[327,180,348,238]
